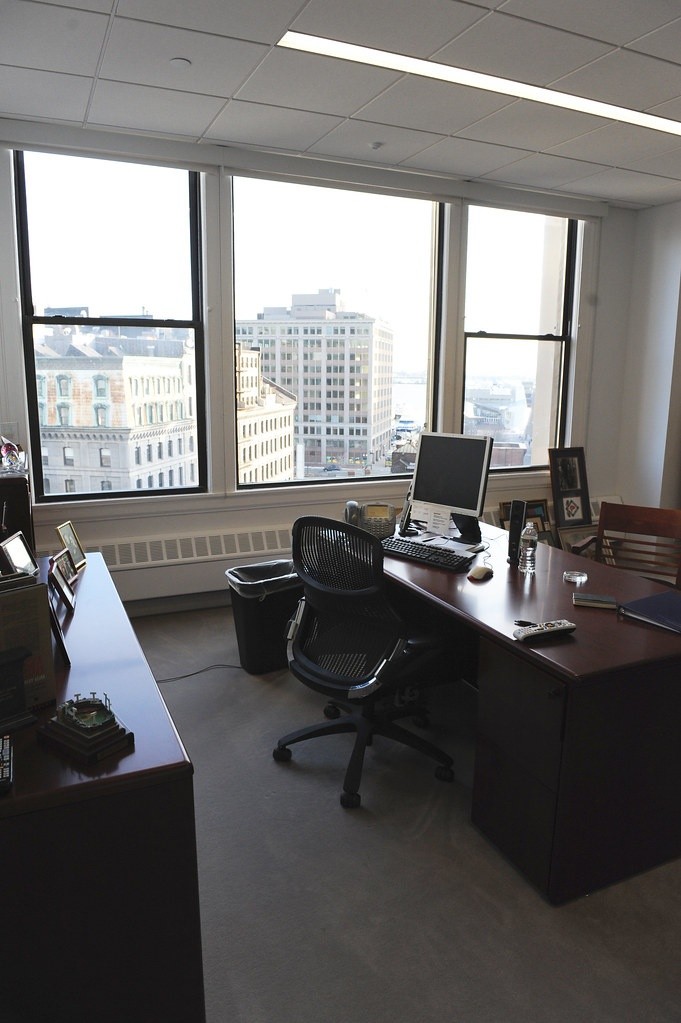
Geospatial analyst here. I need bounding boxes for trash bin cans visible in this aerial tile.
[224,558,305,675]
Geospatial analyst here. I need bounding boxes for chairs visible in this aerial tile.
[272,516,455,811]
[571,501,681,587]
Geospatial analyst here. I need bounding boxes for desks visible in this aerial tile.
[0,551,207,1023]
[314,518,681,908]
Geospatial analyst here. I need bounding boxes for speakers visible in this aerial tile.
[398,482,418,537]
[507,499,527,565]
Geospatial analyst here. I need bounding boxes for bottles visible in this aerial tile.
[517,521,538,573]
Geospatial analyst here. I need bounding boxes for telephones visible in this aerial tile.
[343,501,396,540]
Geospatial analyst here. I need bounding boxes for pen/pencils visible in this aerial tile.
[514,619,537,626]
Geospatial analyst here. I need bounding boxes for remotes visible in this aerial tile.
[513,619,577,642]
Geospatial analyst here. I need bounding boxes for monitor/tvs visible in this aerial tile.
[410,431,495,554]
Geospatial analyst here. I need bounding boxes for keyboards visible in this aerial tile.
[380,536,477,570]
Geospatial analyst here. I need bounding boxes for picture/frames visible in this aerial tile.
[536,531,556,548]
[0,531,40,576]
[55,520,87,568]
[48,548,78,586]
[558,523,616,566]
[48,562,76,611]
[548,446,591,525]
[500,499,549,525]
[499,514,546,535]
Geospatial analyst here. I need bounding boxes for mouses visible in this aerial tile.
[466,566,493,581]
